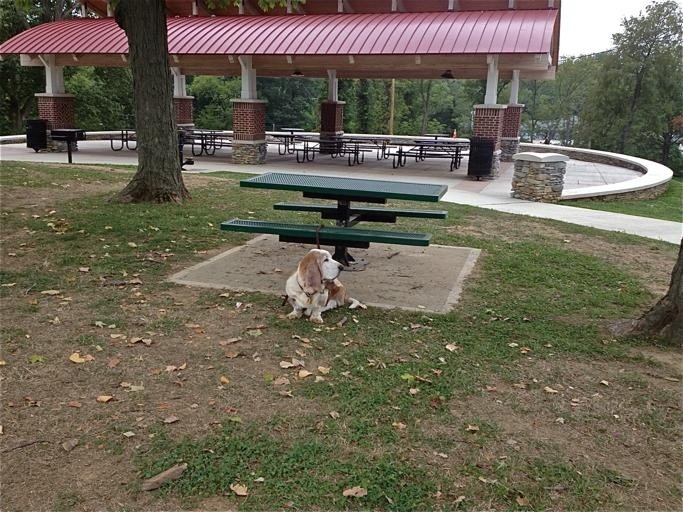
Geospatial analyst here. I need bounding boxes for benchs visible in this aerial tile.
[107,122,471,172]
[217,200,449,273]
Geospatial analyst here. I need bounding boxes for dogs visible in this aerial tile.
[285,248,347,325]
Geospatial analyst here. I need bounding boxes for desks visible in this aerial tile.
[238,172,460,273]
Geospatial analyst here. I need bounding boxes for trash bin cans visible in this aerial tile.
[27,119,46,153]
[467,138,490,181]
